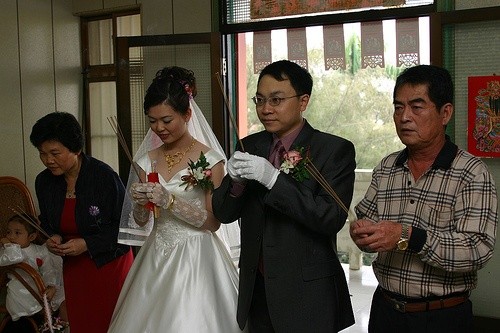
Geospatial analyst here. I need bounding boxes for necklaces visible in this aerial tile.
[163,136,196,179]
[64,158,80,199]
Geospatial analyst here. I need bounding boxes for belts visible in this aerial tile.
[256,276,264,286]
[378,287,469,313]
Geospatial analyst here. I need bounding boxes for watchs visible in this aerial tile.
[393,223,409,253]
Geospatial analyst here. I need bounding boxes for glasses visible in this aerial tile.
[251,95,299,106]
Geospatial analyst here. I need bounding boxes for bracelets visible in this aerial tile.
[166,193,175,211]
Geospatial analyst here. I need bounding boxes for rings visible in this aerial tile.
[149,181,157,199]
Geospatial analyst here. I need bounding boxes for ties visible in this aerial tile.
[273,141,285,170]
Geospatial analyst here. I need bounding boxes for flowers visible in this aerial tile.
[88,205,101,228]
[38,317,69,333]
[179,150,215,192]
[281,147,309,182]
[36,258,44,276]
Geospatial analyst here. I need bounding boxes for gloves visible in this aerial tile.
[227,151,281,190]
[127,182,208,229]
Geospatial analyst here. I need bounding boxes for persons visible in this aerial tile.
[349,65,499,333]
[107,65,239,333]
[0,214,65,333]
[29,112,135,333]
[211,60,356,333]
